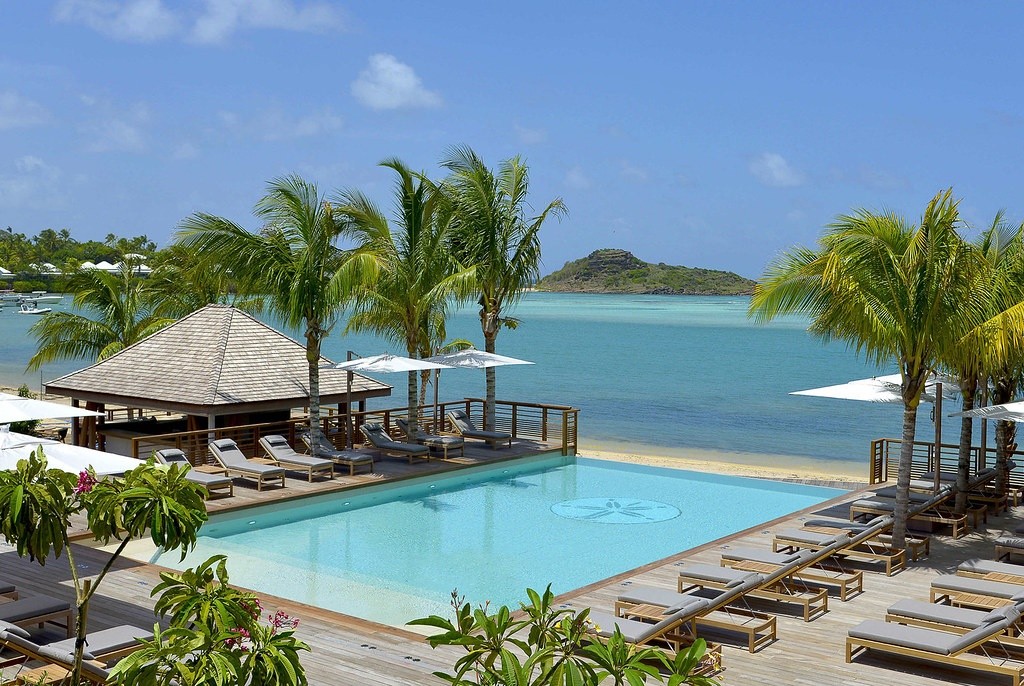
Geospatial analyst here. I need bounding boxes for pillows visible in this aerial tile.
[48,643,94,660]
[0,620,32,638]
[906,504,922,513]
[213,438,237,448]
[450,410,468,419]
[724,572,759,588]
[781,549,812,563]
[866,514,891,526]
[159,449,185,458]
[264,435,287,444]
[975,468,994,476]
[818,533,849,546]
[400,419,418,425]
[1010,590,1024,602]
[661,597,701,615]
[305,431,326,439]
[981,605,1016,622]
[364,423,383,430]
[936,483,954,494]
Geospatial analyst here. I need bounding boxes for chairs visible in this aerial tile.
[37,642,249,686]
[448,410,511,451]
[772,517,906,577]
[0,594,74,639]
[678,544,836,623]
[799,503,930,563]
[720,538,863,602]
[207,439,285,492]
[845,524,1024,686]
[615,575,776,654]
[300,431,374,476]
[567,599,722,686]
[154,449,233,502]
[0,620,155,686]
[258,434,334,483]
[876,460,1024,517]
[44,428,68,444]
[395,419,464,460]
[359,422,430,465]
[850,486,968,540]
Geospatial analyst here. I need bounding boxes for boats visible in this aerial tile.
[17,303,52,314]
[0,287,64,310]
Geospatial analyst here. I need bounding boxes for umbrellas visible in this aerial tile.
[319,349,459,451]
[788,368,1024,498]
[421,346,537,437]
[0,388,165,477]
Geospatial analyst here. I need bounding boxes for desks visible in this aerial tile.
[731,559,794,602]
[799,525,854,551]
[981,572,1024,585]
[16,660,107,686]
[623,603,696,654]
[951,592,1019,610]
[248,457,281,481]
[194,465,232,490]
